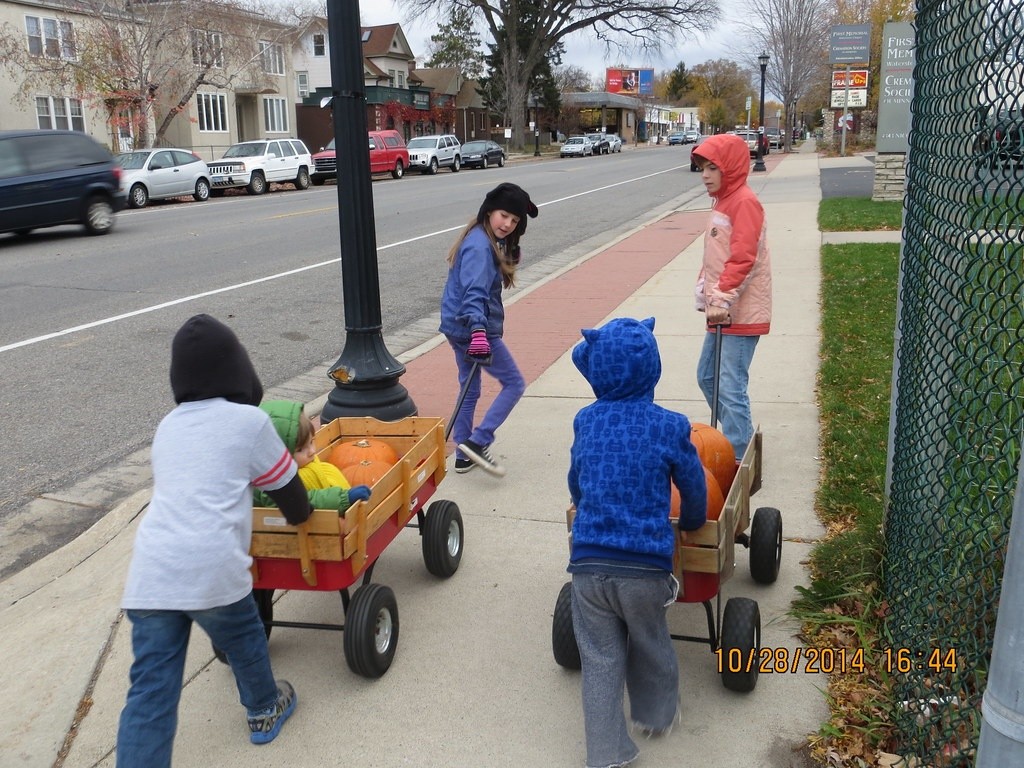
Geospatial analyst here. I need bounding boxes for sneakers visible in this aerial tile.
[455,458,476,473]
[459,438,505,478]
[246,680,297,744]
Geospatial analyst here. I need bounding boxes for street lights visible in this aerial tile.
[800,110,803,127]
[689,112,693,130]
[534,95,541,156]
[792,98,798,144]
[656,109,661,144]
[752,50,771,172]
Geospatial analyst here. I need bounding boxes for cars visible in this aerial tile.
[979,107,1024,170]
[725,127,786,159]
[588,134,622,155]
[461,140,507,169]
[560,136,592,156]
[112,147,211,208]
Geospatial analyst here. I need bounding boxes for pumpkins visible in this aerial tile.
[670,423,736,522]
[342,459,393,490]
[327,438,398,468]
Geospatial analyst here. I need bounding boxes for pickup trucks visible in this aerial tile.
[669,131,688,145]
[686,131,699,143]
[312,129,409,186]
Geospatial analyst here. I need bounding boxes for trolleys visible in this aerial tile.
[210,348,494,682]
[550,311,785,693]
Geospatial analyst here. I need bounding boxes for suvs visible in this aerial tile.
[405,133,463,175]
[207,137,315,198]
[0,129,125,235]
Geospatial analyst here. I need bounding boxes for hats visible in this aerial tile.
[478,182,538,243]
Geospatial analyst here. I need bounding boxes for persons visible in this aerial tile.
[691,134,772,481]
[250,398,370,511]
[116,314,312,768]
[565,318,708,768]
[436,181,539,478]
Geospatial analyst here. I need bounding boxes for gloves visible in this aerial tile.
[346,486,372,503]
[468,330,491,359]
